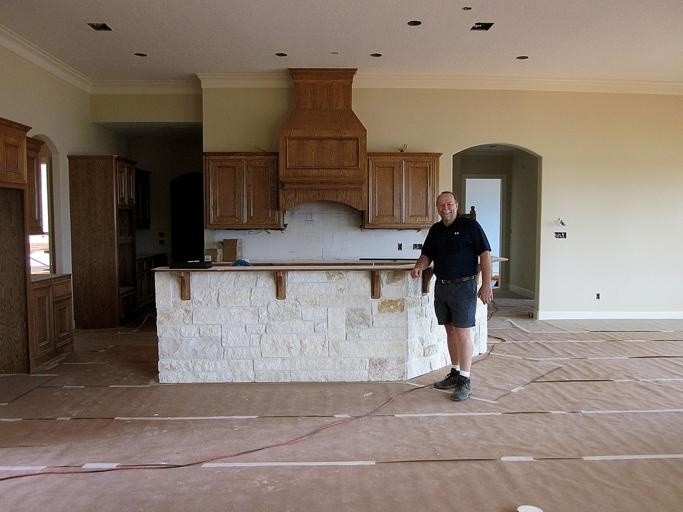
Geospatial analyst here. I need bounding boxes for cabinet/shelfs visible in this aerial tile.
[0,117,28,194]
[135,166,153,230]
[364,152,443,230]
[67,152,140,329]
[27,135,46,236]
[202,149,287,231]
[136,252,168,306]
[30,274,75,372]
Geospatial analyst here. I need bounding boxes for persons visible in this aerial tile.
[411,192,494,401]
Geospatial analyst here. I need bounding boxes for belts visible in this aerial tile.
[434,275,476,284]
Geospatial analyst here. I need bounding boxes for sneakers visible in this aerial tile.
[450,376,471,401]
[432,368,460,389]
